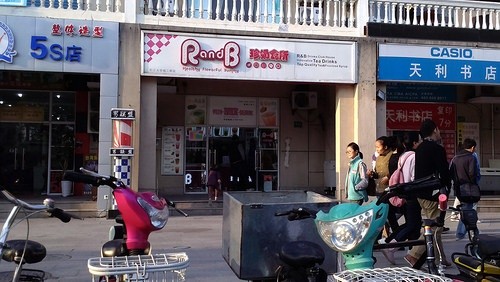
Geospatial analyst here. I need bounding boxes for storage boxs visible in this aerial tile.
[221,190,338,280]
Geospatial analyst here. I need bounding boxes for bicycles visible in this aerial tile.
[0,180,68,282]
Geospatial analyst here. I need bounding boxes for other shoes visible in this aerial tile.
[404,254,418,267]
[442,226,449,232]
[214,197,217,202]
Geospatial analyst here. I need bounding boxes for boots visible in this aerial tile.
[378,238,403,265]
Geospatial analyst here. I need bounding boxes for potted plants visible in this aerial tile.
[59,161,72,197]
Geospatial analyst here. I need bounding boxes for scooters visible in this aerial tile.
[69,168,193,282]
[274,172,500,282]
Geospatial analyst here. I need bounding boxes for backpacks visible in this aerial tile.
[388,169,407,208]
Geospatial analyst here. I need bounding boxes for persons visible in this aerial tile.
[371,120,452,269]
[345,142,368,206]
[207,139,255,207]
[448,138,482,242]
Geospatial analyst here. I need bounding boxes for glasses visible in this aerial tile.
[345,151,356,154]
